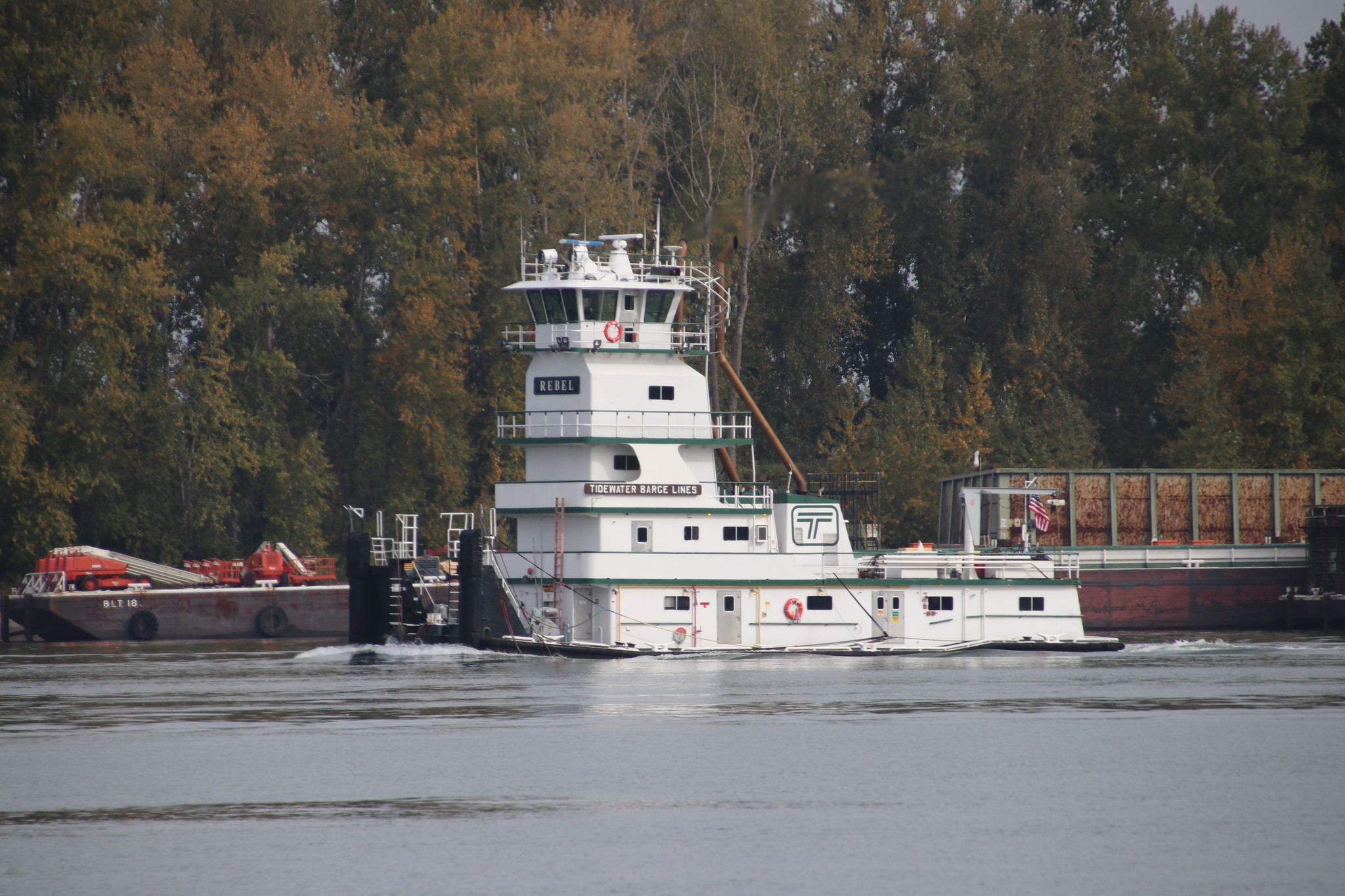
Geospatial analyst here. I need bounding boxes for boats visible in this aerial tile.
[473,217,1128,657]
[0,544,461,643]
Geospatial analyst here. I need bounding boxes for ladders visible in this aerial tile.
[554,498,563,632]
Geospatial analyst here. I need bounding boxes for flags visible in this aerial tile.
[1028,484,1050,532]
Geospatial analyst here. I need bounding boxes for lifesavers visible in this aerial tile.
[604,321,622,343]
[255,605,288,636]
[129,610,158,640]
[784,598,803,619]
[531,326,535,340]
